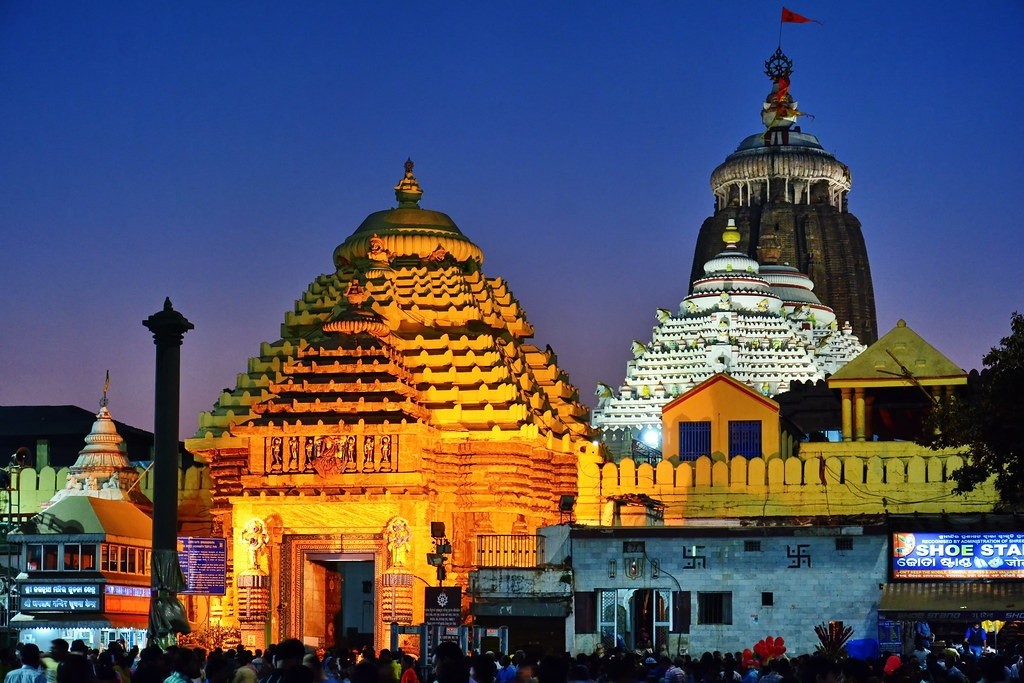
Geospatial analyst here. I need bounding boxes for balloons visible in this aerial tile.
[887,655,901,669]
[753,636,787,657]
[742,649,752,660]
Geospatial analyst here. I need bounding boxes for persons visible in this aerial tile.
[134,645,206,683]
[306,439,313,463]
[290,437,299,460]
[272,437,282,464]
[42,638,69,683]
[432,642,537,683]
[381,438,390,462]
[57,639,97,683]
[2,643,46,683]
[86,639,139,683]
[365,438,374,461]
[320,645,421,683]
[538,643,842,683]
[844,616,1024,683]
[204,638,322,683]
[347,436,355,463]
[15,642,25,657]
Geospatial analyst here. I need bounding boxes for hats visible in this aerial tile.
[645,656,657,664]
[679,648,689,656]
[942,648,961,663]
[302,653,317,666]
[70,639,86,651]
[882,655,903,672]
